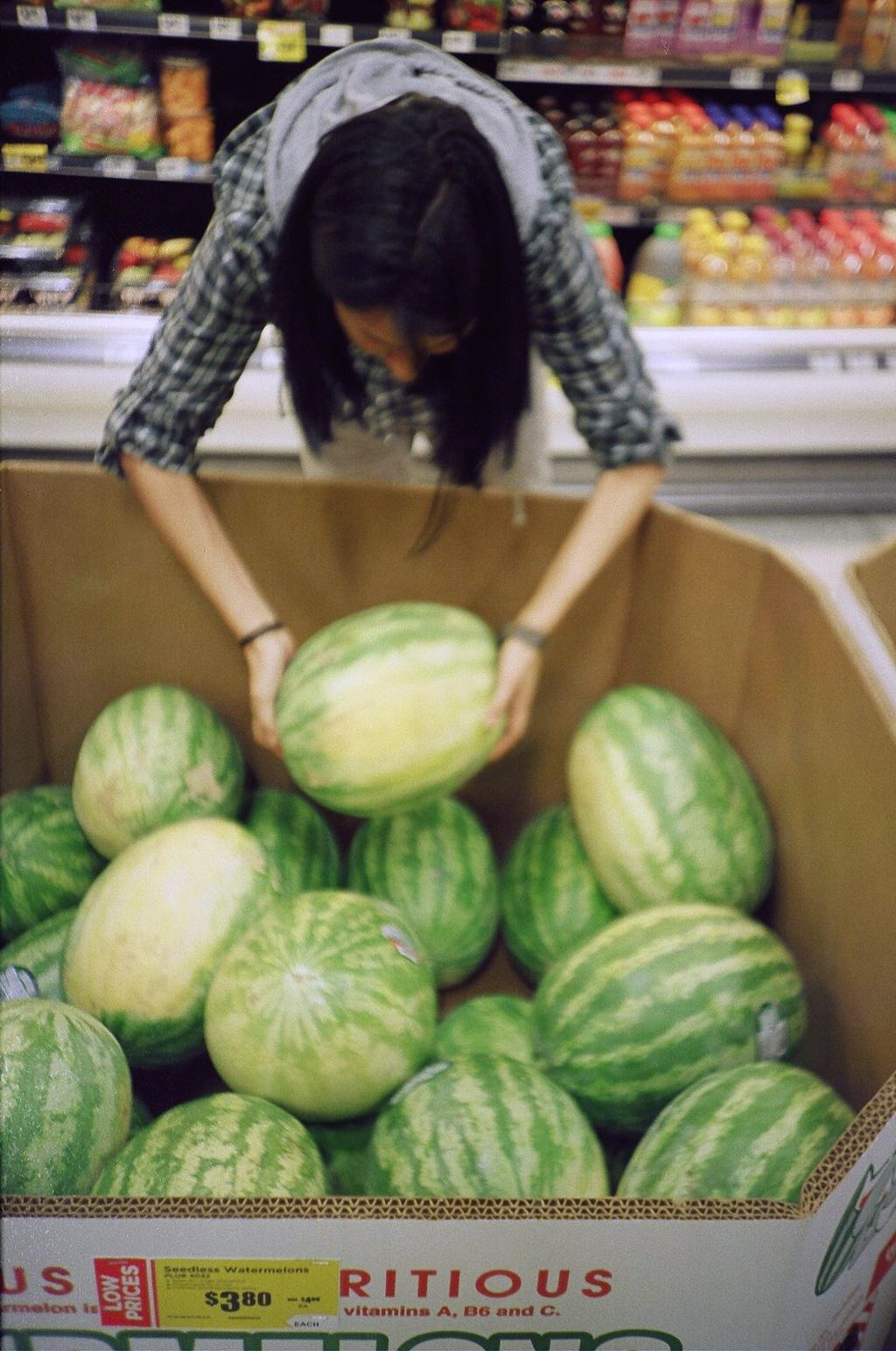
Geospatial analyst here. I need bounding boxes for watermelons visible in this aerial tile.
[0,683,860,1206]
[271,599,507,815]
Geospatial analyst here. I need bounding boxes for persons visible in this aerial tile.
[94,34,682,768]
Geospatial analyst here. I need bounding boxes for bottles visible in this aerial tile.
[528,86,896,333]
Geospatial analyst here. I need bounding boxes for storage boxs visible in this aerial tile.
[0,459,896,1351]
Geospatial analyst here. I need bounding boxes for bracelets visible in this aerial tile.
[238,621,286,649]
[504,620,547,650]
